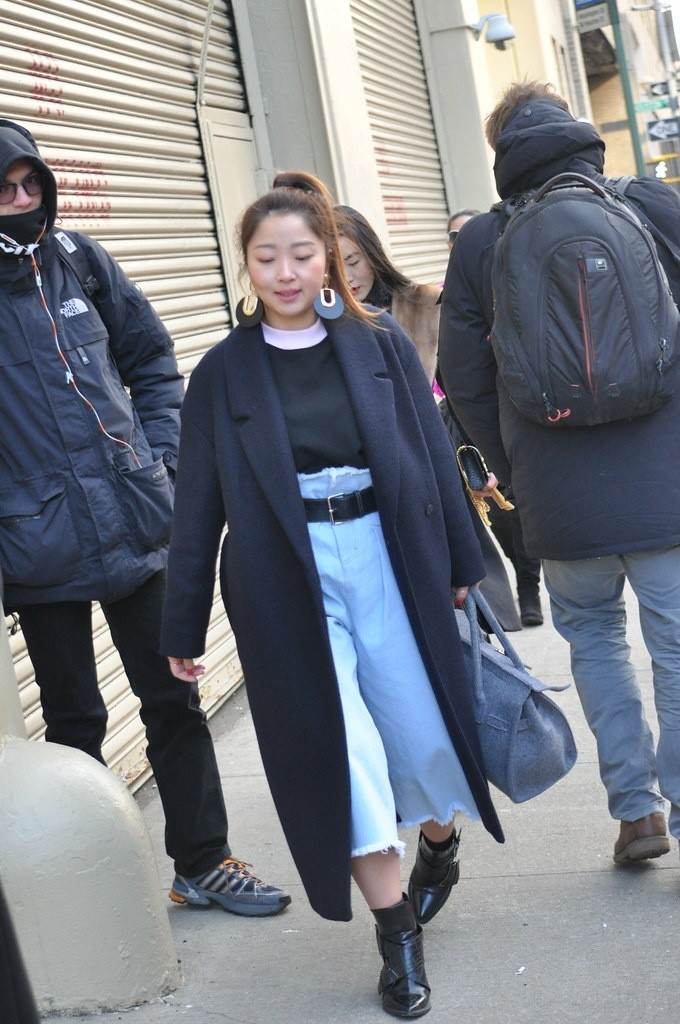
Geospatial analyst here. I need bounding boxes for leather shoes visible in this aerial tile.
[614,814,670,865]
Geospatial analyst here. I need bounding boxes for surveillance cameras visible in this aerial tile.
[485,14,516,51]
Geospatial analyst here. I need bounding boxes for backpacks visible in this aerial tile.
[488,173,680,428]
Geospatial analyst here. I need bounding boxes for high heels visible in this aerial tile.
[370,894,432,1018]
[408,828,461,926]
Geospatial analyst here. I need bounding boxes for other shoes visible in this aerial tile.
[518,587,544,625]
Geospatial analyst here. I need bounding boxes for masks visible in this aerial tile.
[0,204,49,248]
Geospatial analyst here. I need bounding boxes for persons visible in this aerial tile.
[162,171,506,1019]
[446,209,544,626]
[333,206,524,632]
[0,118,292,917]
[436,82,680,864]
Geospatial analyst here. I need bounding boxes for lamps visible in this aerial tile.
[472,9,520,51]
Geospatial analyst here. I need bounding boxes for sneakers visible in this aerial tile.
[169,857,292,918]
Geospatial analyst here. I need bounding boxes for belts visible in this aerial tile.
[301,486,377,525]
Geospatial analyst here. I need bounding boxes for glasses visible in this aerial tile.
[0,173,43,204]
[447,229,460,249]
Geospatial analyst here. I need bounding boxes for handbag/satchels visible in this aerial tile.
[449,587,578,803]
